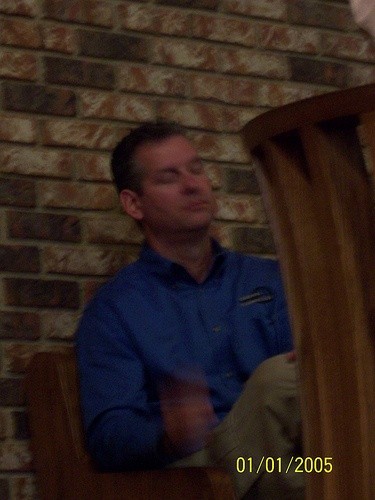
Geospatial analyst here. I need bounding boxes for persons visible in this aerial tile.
[72,121,308,474]
